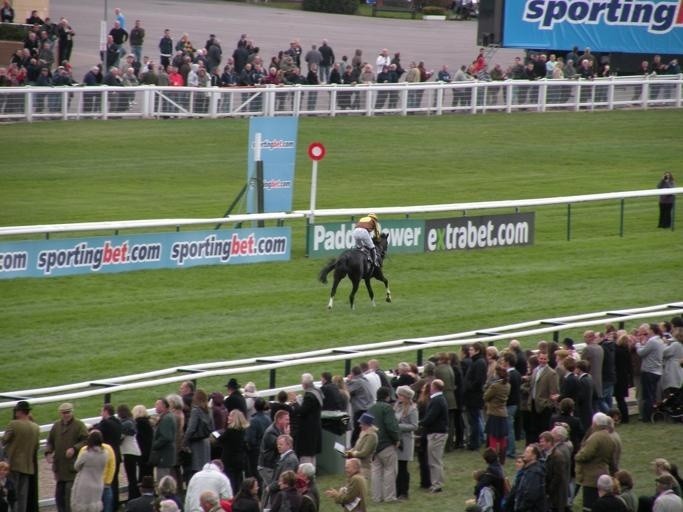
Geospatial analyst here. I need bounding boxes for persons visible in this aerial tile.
[657,170,677,229]
[632,55,680,105]
[220,33,610,119]
[448,1,479,21]
[320,314,683,511]
[0,373,324,512]
[82,7,222,115]
[1,1,79,119]
[352,210,383,268]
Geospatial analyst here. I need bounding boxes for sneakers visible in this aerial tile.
[369,482,443,502]
[445,439,486,452]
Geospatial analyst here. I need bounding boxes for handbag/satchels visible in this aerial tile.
[182,408,209,442]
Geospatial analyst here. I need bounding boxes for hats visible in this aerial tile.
[397,384,415,399]
[56,403,73,412]
[13,400,32,410]
[560,338,573,349]
[223,378,241,389]
[654,474,675,485]
[356,413,376,426]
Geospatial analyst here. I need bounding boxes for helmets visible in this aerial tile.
[367,213,378,220]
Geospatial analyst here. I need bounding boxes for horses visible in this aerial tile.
[319,232,392,309]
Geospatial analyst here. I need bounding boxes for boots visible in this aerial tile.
[370,247,378,268]
[498,448,506,465]
